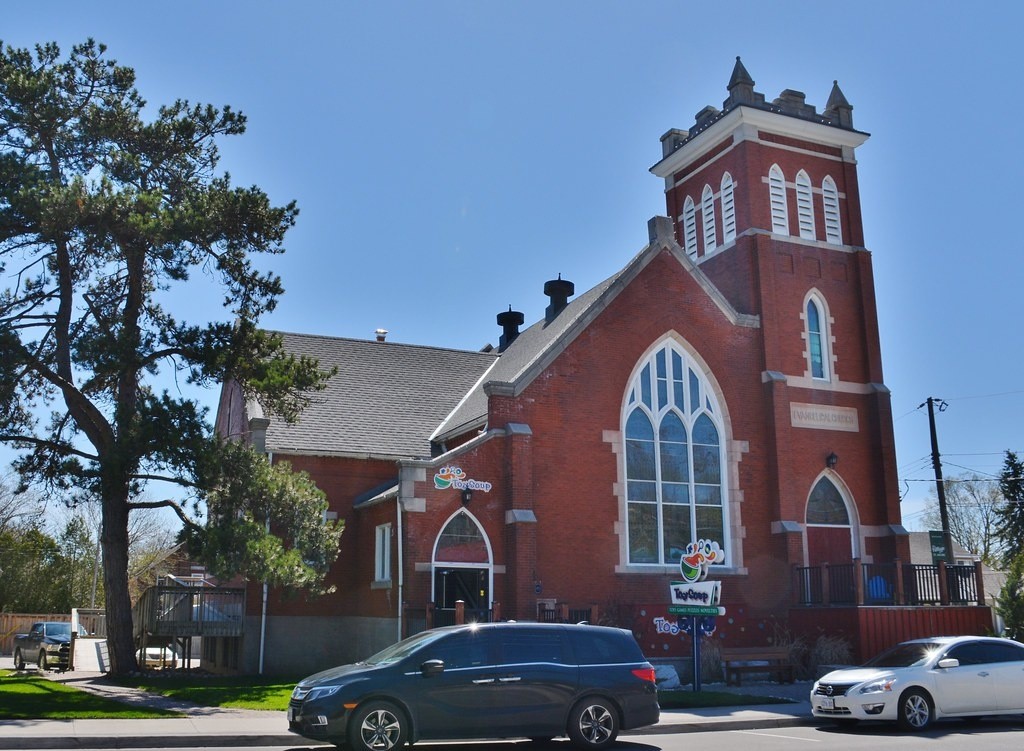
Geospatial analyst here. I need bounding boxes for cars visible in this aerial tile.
[136,647,179,668]
[810,634,1023,733]
[286,618,661,751]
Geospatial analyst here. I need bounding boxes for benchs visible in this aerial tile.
[718,647,797,687]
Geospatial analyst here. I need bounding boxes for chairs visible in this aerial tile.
[868,575,892,605]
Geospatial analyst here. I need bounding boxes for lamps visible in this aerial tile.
[462,484,472,506]
[827,452,837,468]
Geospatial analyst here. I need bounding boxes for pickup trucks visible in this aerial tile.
[11,621,96,671]
